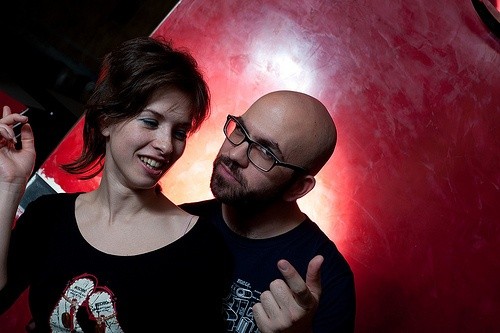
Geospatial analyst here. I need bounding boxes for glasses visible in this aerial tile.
[223,114,308,174]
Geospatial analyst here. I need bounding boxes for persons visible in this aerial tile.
[177,90,357,333]
[1,37,222,333]
